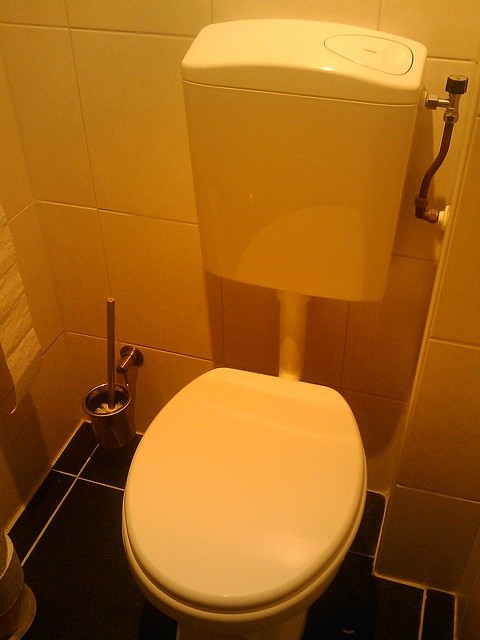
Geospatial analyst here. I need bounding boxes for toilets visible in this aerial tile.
[118,17,429,639]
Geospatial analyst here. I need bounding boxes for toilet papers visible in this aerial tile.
[1,207,46,416]
